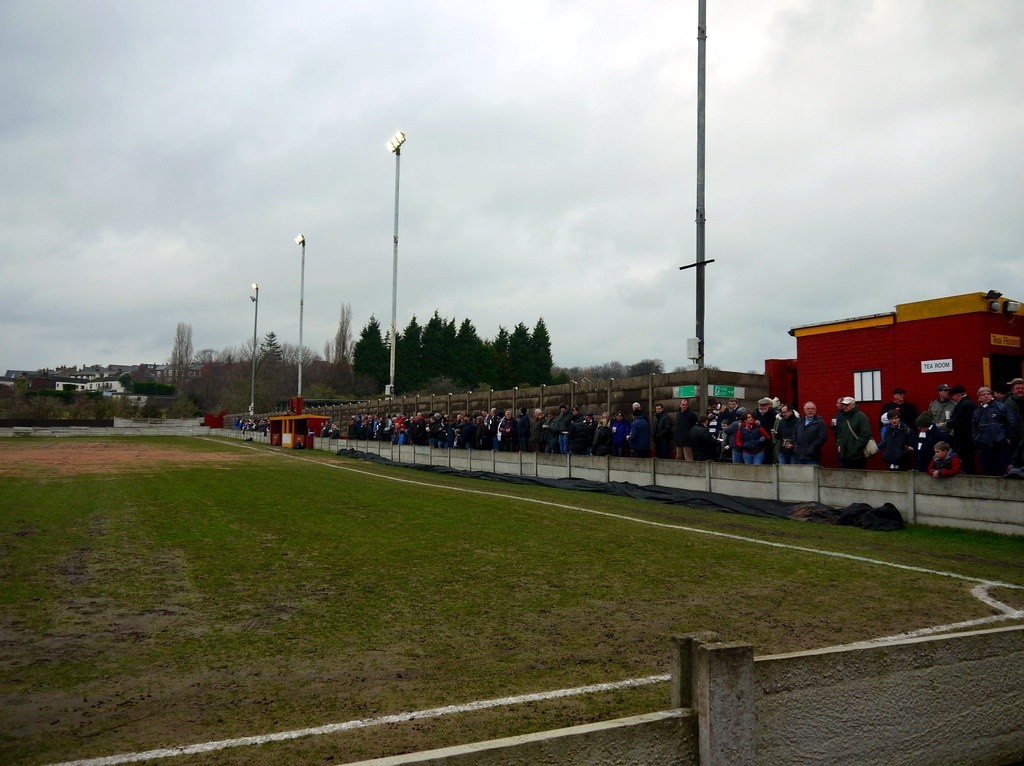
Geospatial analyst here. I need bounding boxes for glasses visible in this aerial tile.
[681,404,687,406]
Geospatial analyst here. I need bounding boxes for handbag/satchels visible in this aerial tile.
[862,437,880,457]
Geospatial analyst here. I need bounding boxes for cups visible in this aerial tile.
[832,419,837,425]
[946,411,951,419]
[613,427,616,432]
[426,427,429,431]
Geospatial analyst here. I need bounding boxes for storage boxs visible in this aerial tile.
[309,432,315,436]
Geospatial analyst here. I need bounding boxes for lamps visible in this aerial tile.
[990,300,1001,313]
[1002,301,1021,314]
[982,290,1003,300]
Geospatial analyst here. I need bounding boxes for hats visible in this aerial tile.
[881,412,891,424]
[772,397,781,409]
[559,403,567,408]
[758,398,769,404]
[841,397,855,404]
[1007,378,1023,384]
[585,411,594,415]
[893,388,905,393]
[616,411,624,417]
[915,411,932,427]
[938,384,952,390]
[736,407,746,417]
[949,385,965,395]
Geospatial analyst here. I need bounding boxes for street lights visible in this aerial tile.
[248,282,259,415]
[386,130,407,401]
[294,233,306,396]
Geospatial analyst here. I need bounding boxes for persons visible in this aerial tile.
[830,397,871,469]
[652,399,698,462]
[876,383,978,477]
[321,419,340,439]
[689,397,828,465]
[234,416,270,433]
[348,412,454,449]
[454,409,554,453]
[553,402,650,459]
[972,378,1024,476]
